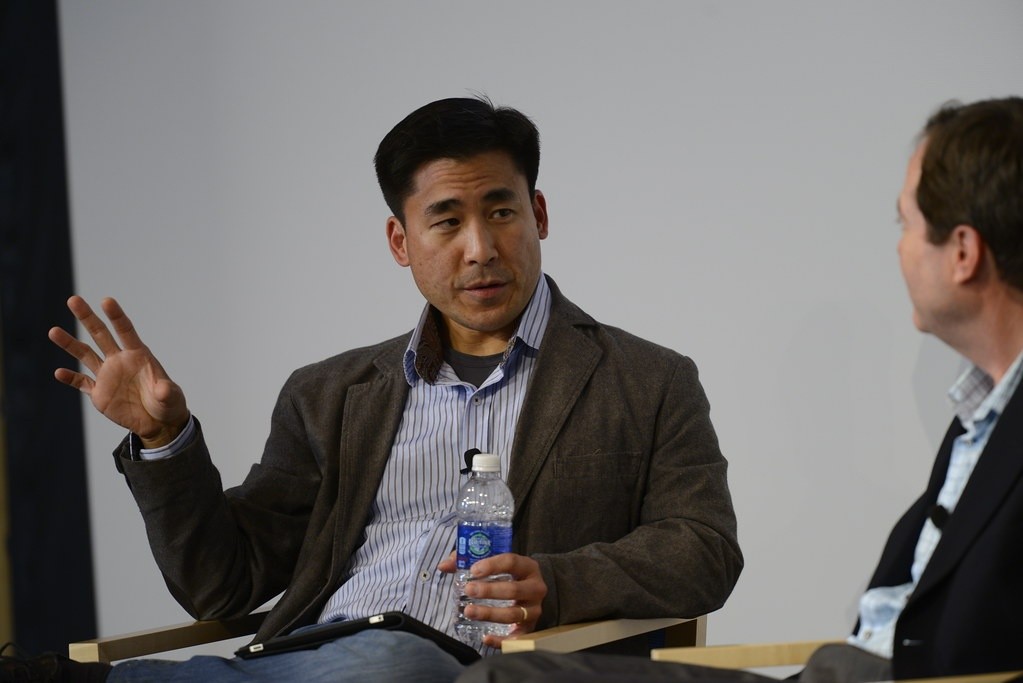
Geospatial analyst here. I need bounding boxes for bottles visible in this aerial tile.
[452,451,515,642]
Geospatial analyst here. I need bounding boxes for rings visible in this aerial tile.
[515,604,529,625]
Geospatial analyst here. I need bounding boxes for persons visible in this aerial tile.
[455,97,1022,683]
[0,95,747,683]
